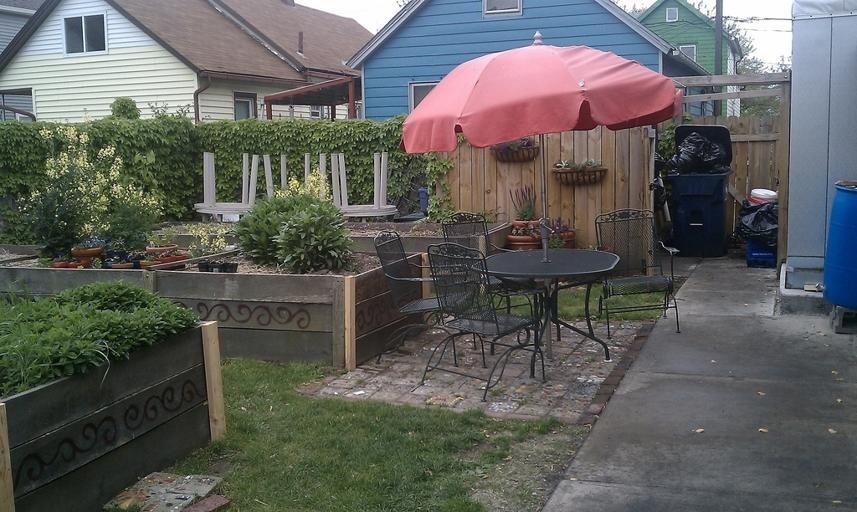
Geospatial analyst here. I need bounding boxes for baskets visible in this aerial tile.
[552,169,608,185]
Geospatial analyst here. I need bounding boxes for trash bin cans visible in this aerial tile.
[668,125,733,258]
[742,199,779,268]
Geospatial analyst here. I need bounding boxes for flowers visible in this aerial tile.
[551,159,602,169]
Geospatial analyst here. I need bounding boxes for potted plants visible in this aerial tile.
[52,227,190,268]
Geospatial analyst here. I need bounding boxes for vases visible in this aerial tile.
[553,168,607,183]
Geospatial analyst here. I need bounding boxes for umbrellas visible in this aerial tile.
[403,29,684,357]
